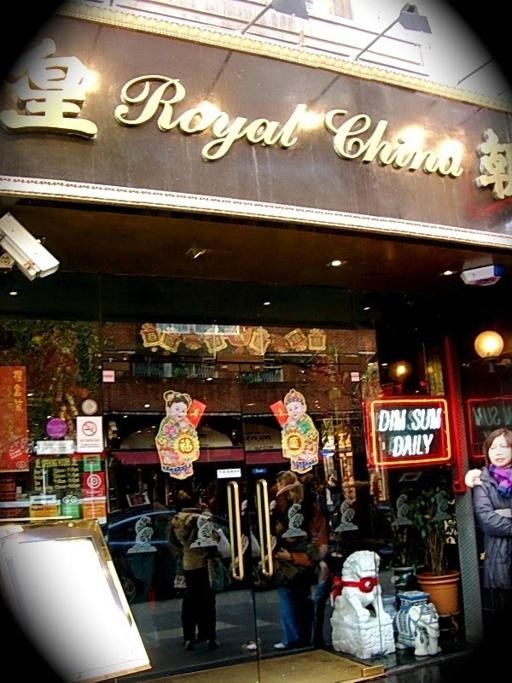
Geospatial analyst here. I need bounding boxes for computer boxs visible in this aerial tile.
[0,212,61,281]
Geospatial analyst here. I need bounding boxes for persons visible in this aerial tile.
[280,389,319,473]
[173,491,221,652]
[154,390,200,479]
[474,427,512,683]
[274,473,391,648]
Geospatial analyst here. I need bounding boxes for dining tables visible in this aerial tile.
[389,486,460,614]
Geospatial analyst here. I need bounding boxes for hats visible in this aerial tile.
[106,509,272,604]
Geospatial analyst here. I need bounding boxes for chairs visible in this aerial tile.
[183,638,221,650]
[273,642,290,649]
